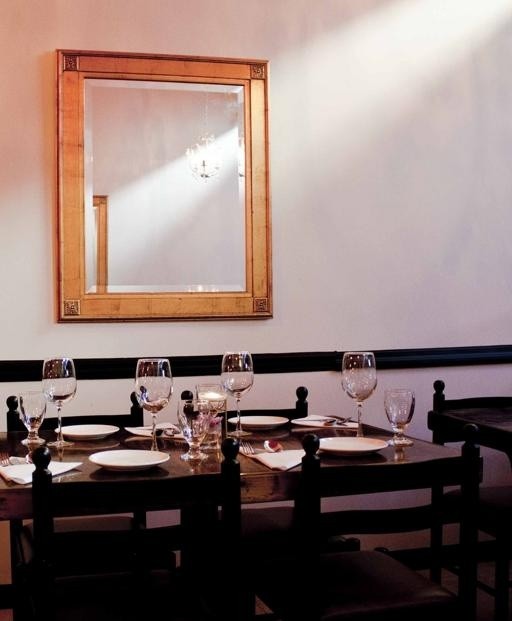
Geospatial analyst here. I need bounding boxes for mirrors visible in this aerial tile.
[52,49,277,320]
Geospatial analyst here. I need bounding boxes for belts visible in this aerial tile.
[134,357,173,456]
[176,399,212,462]
[16,389,48,446]
[383,388,415,447]
[195,382,227,450]
[340,351,378,436]
[220,350,255,438]
[42,357,78,447]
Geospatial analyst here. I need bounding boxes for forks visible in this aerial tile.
[0,452,14,468]
[241,441,260,457]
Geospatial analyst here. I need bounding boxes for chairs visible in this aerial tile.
[0,379,511,619]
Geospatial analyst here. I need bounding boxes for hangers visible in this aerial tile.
[262,439,283,453]
[294,415,352,426]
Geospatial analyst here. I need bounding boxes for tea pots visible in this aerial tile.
[52,49,277,320]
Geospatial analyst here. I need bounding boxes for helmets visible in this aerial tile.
[54,424,121,441]
[88,449,171,472]
[228,415,290,431]
[317,436,389,457]
